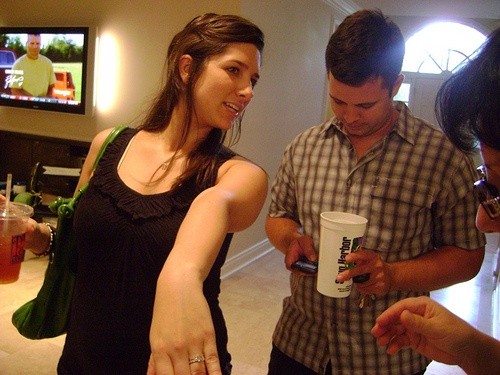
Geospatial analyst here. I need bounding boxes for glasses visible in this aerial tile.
[472,165,499,219]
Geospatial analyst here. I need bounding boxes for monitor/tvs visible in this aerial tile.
[0,25,97,116]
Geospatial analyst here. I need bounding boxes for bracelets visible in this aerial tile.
[32,223,56,257]
[189,354,204,364]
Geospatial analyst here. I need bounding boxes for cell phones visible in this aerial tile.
[291,256,318,274]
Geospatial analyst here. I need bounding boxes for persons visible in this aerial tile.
[10,34,56,98]
[265,10,487,375]
[371,26,500,375]
[0,14,268,375]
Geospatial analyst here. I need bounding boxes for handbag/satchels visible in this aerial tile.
[11,125,129,341]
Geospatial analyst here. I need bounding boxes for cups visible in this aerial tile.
[316,211,368,298]
[0,202,33,285]
